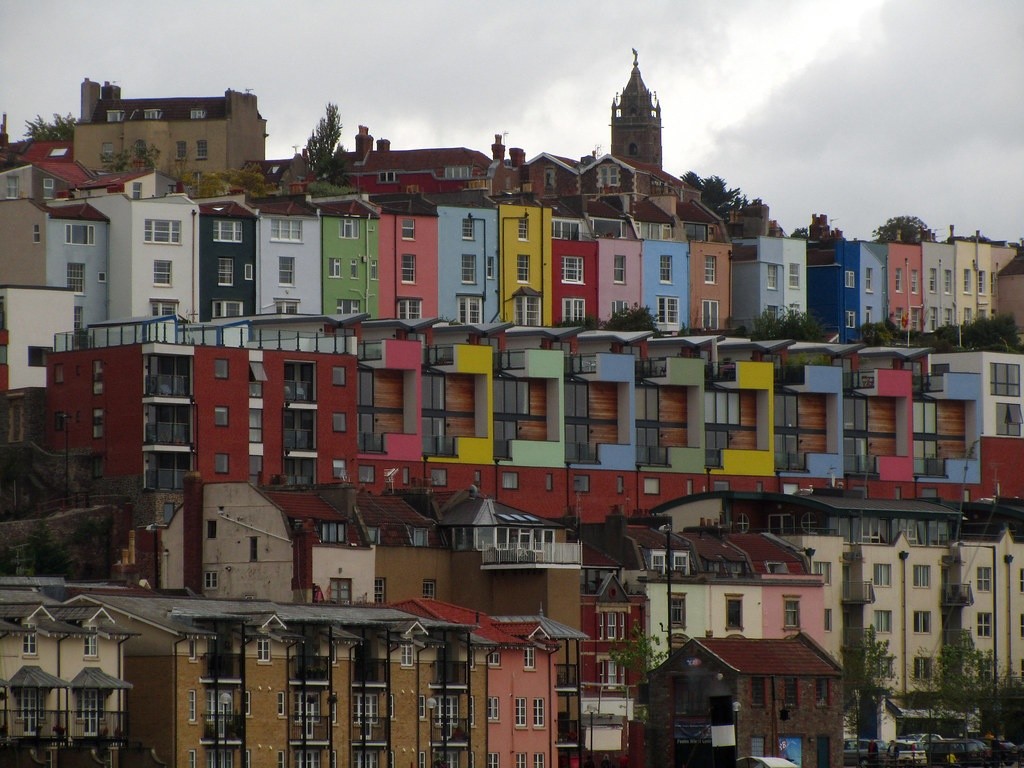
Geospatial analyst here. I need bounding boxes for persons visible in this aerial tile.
[868,737,878,757]
[886,739,899,758]
[984,731,994,739]
[947,751,957,764]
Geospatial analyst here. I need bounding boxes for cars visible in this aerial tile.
[732,755,798,768]
[842,729,1024,768]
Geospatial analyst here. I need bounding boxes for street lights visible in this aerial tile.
[587,703,598,767]
[426,697,437,768]
[732,701,741,768]
[220,692,231,768]
